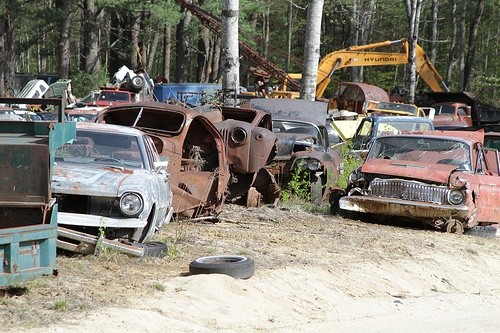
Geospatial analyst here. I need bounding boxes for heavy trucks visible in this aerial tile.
[423,90,500,127]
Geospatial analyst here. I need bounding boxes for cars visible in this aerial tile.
[0,63,500,289]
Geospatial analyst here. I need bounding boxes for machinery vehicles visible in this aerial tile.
[268,36,451,99]
